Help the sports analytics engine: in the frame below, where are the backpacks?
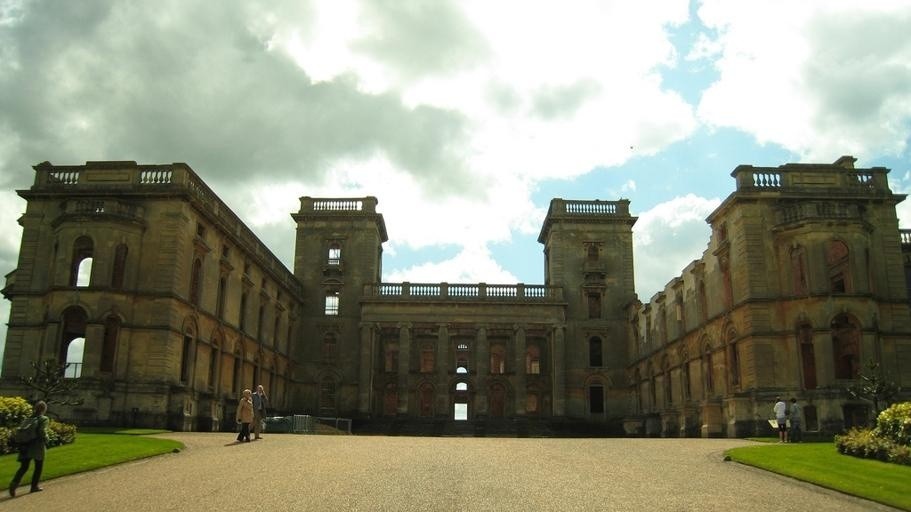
[14,415,39,443]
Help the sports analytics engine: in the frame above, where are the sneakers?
[10,482,17,497]
[31,486,45,492]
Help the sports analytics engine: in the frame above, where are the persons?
[249,384,269,439]
[236,389,254,442]
[790,396,803,442]
[8,401,52,497]
[774,396,788,443]
